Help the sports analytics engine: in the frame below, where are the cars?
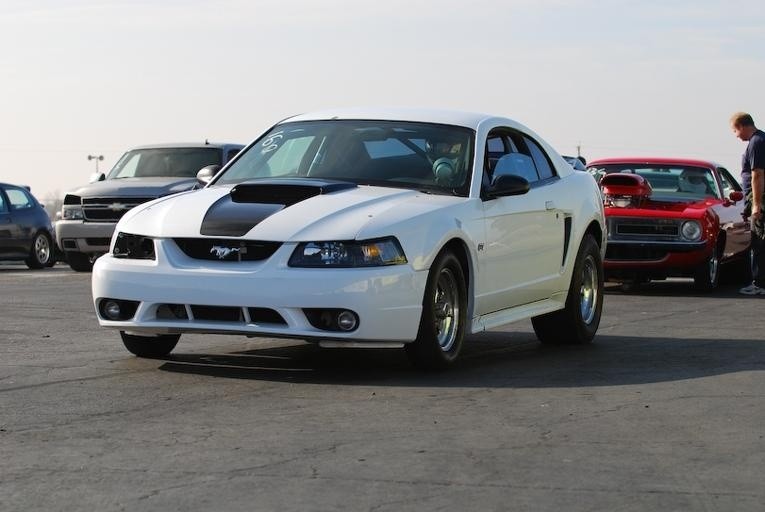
[0,182,53,269]
[55,139,251,272]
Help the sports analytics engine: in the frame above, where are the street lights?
[88,155,104,173]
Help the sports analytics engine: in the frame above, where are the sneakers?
[738,283,765,297]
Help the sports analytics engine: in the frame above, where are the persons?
[423,135,462,182]
[676,167,708,194]
[730,109,765,298]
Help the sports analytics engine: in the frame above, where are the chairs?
[676,169,717,196]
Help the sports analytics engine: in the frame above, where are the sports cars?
[91,110,610,372]
[586,156,752,289]
